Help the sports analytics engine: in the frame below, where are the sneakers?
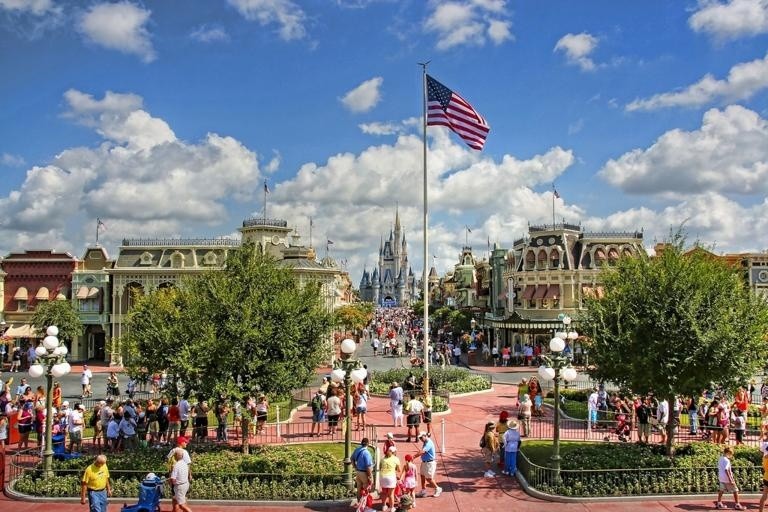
[415,492,427,497]
[485,470,515,477]
[734,503,747,510]
[361,428,366,430]
[413,502,416,507]
[382,505,388,511]
[309,431,337,437]
[355,429,359,431]
[364,507,376,512]
[427,433,431,437]
[406,438,411,442]
[416,439,419,442]
[390,507,396,512]
[434,487,442,497]
[190,437,230,445]
[634,439,667,445]
[91,443,176,448]
[716,504,727,508]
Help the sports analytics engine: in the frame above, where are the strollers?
[603,417,629,442]
[120,476,168,512]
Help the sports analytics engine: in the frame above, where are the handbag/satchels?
[479,436,486,448]
[517,412,524,420]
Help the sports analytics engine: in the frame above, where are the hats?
[498,411,508,420]
[405,454,413,462]
[79,404,85,411]
[384,432,393,438]
[389,446,396,453]
[63,401,69,406]
[417,431,426,437]
[507,420,520,429]
[259,393,266,398]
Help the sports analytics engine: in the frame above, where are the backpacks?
[89,414,98,426]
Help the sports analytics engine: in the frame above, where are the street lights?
[537,338,577,487]
[554,317,579,387]
[330,339,368,494]
[26,325,72,482]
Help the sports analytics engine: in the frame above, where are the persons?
[169,450,192,512]
[166,436,192,512]
[759,442,768,512]
[81,454,113,512]
[715,445,747,511]
[587,379,768,447]
[0,343,270,453]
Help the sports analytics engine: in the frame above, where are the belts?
[357,470,366,472]
[422,460,433,462]
[91,489,106,492]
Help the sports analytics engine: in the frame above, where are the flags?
[554,185,560,199]
[265,182,271,194]
[466,225,473,234]
[327,239,334,244]
[426,73,490,152]
[96,219,107,235]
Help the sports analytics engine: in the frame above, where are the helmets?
[400,495,413,504]
